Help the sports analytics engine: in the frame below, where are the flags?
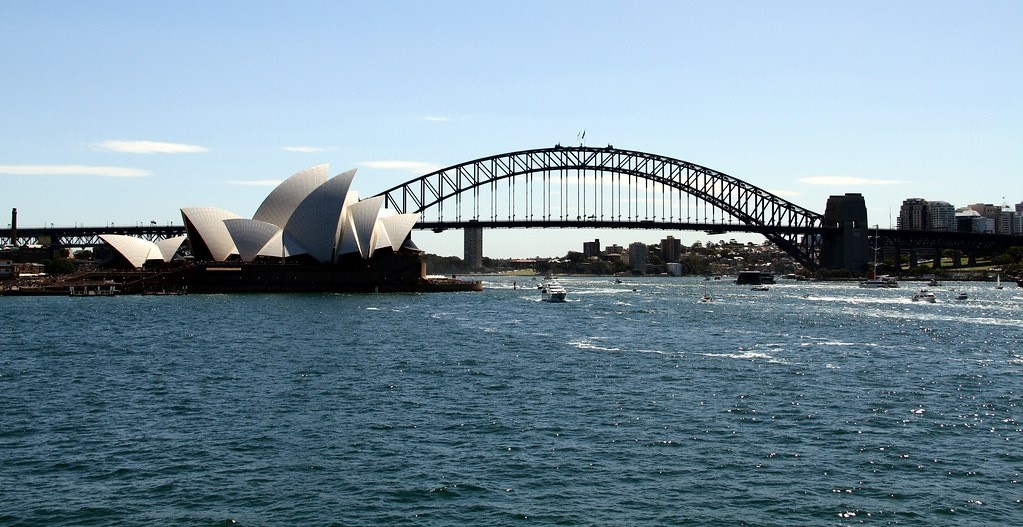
[582,130,585,139]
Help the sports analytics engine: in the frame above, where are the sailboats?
[859,224,901,288]
[995,274,1003,288]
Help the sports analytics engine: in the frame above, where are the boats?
[928,279,942,287]
[751,284,769,292]
[955,293,968,299]
[736,271,776,284]
[616,277,622,283]
[700,280,716,305]
[542,279,568,301]
[913,289,936,303]
